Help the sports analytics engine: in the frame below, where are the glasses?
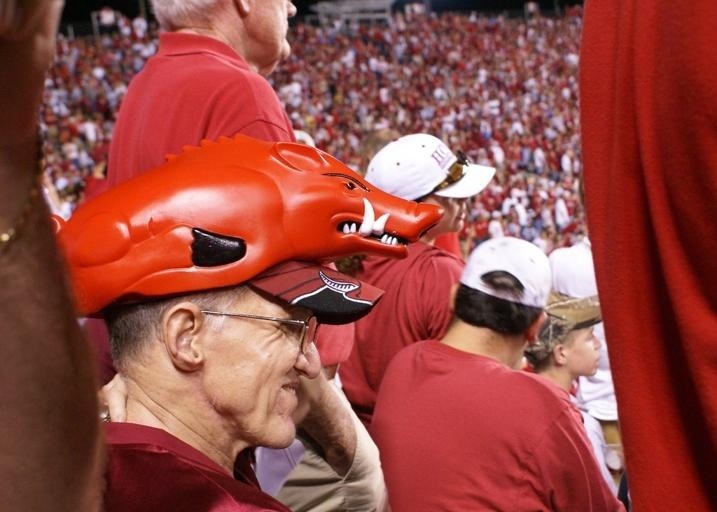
[421,149,471,201]
[202,312,321,354]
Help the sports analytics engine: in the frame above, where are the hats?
[364,132,497,201]
[249,261,385,326]
[459,236,552,308]
[524,292,602,366]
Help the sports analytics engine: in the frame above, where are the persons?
[0,0,716,512]
